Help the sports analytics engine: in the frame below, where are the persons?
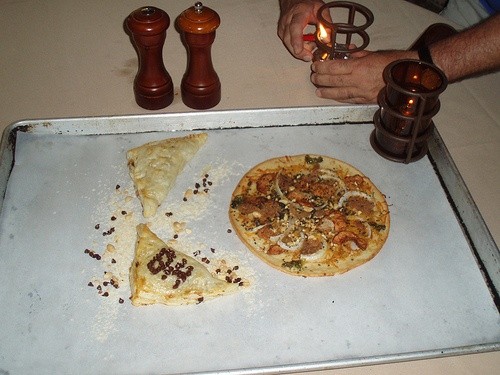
[277,0,500,104]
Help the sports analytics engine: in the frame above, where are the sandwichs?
[126,133,208,219]
[128,223,239,308]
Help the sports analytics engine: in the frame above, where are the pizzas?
[227,154,391,277]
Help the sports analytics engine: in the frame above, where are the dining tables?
[3,0,500,375]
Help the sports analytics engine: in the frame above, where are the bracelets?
[416,41,433,64]
